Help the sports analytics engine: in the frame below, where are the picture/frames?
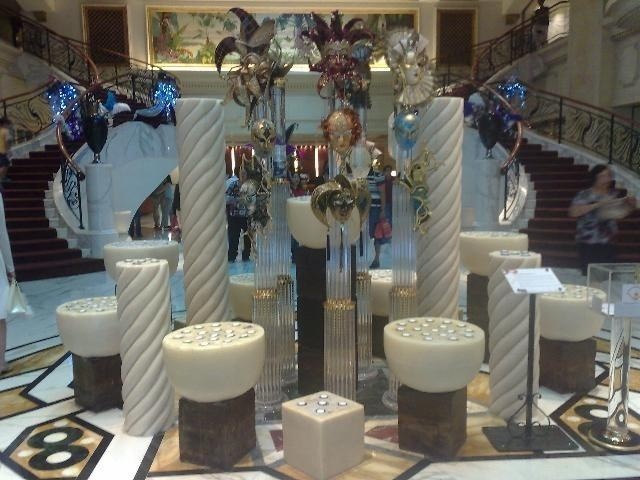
[144,5,421,68]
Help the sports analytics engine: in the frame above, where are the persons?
[0,194,15,373]
[8,0,23,49]
[130,162,394,270]
[0,155,11,181]
[567,163,634,274]
[0,118,13,154]
[530,0,549,49]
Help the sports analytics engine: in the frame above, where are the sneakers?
[369,259,382,269]
[0,365,14,375]
[153,225,179,232]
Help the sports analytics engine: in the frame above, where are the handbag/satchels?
[596,184,635,221]
[7,279,36,318]
[374,218,393,240]
